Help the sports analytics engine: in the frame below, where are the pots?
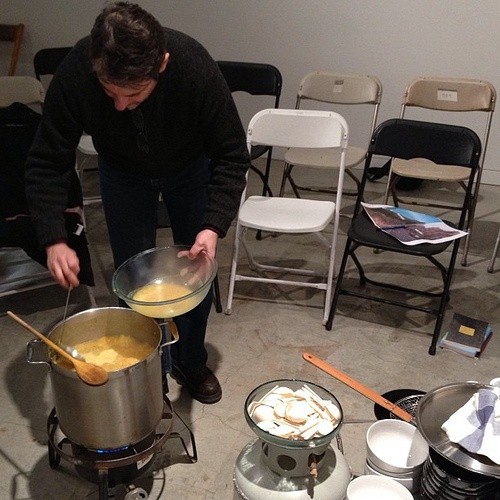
[26,308,178,451]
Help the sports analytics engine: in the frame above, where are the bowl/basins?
[244,378,345,449]
[345,419,430,500]
[113,244,218,319]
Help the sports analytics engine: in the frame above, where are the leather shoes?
[170,363,222,404]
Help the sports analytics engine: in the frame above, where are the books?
[439,313,492,360]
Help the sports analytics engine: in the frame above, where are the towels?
[441,377,500,467]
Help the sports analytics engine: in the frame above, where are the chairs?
[273,70,383,238]
[326,120,482,354]
[371,76,496,264]
[224,108,350,323]
[0,23,103,310]
[216,60,282,240]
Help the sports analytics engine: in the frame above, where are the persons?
[22,2,251,404]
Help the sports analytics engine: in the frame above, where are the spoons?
[248,382,340,442]
